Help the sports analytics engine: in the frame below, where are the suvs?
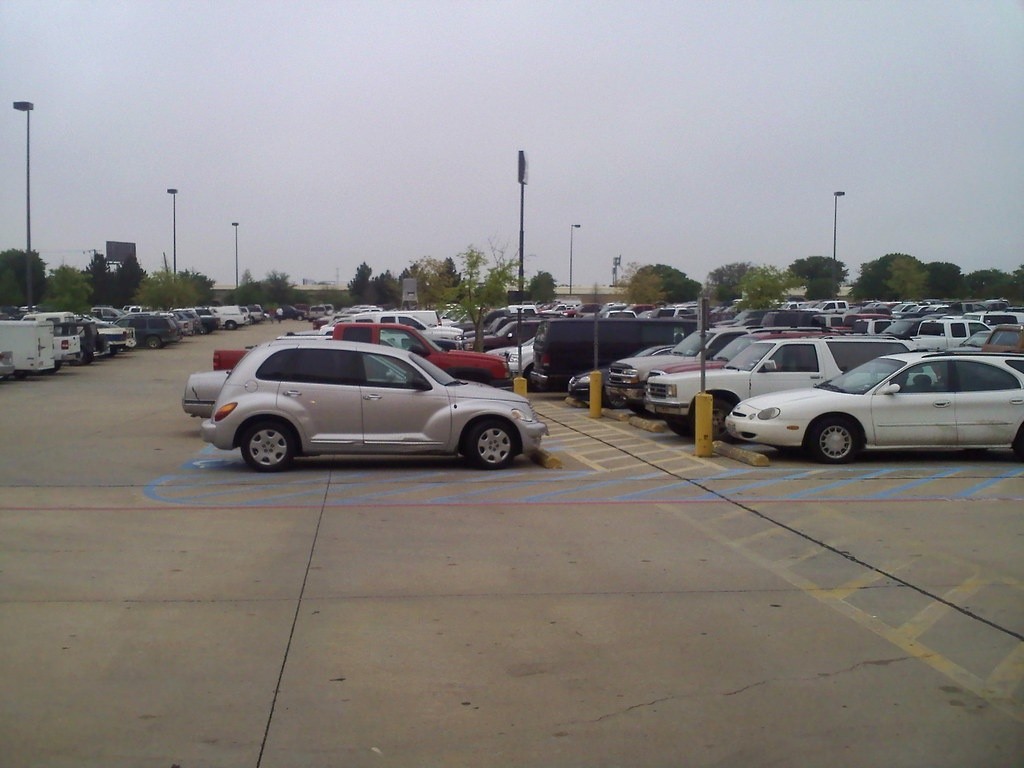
[176,297,1023,389]
[112,311,183,350]
[605,324,849,416]
[646,325,851,381]
[17,311,138,372]
[170,312,194,337]
[723,350,1024,463]
[651,328,938,443]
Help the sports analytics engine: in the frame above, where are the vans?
[91,307,126,323]
[125,305,164,314]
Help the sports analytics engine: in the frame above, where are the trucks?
[0,320,55,378]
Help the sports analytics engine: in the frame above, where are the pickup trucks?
[213,323,514,394]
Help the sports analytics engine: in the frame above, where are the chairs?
[912,374,930,386]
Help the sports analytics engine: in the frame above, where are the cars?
[182,352,418,419]
[0,350,17,376]
[567,344,677,400]
[202,338,550,473]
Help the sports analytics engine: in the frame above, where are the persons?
[269,309,275,324]
[276,306,283,324]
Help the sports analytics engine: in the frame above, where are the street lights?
[231,222,239,288]
[166,188,178,282]
[570,224,581,299]
[12,100,35,314]
[832,190,848,261]
